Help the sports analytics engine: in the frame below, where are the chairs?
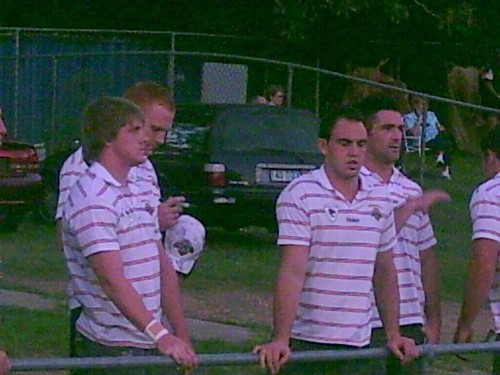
[401,129,431,158]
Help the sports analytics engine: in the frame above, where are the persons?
[364,101,452,375]
[451,124,500,375]
[55,81,200,375]
[251,106,422,375]
[402,95,455,182]
[0,108,8,150]
[254,87,286,106]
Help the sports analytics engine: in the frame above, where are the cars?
[0,139,43,234]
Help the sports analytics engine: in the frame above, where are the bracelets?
[144,317,170,344]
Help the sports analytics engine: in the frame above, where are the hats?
[164,215,205,274]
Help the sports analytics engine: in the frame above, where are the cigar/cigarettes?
[168,196,190,208]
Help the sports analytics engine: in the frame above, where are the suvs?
[37,104,326,238]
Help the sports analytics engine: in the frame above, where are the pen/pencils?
[161,198,197,208]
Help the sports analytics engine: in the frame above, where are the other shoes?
[436,160,444,168]
[443,176,452,180]
